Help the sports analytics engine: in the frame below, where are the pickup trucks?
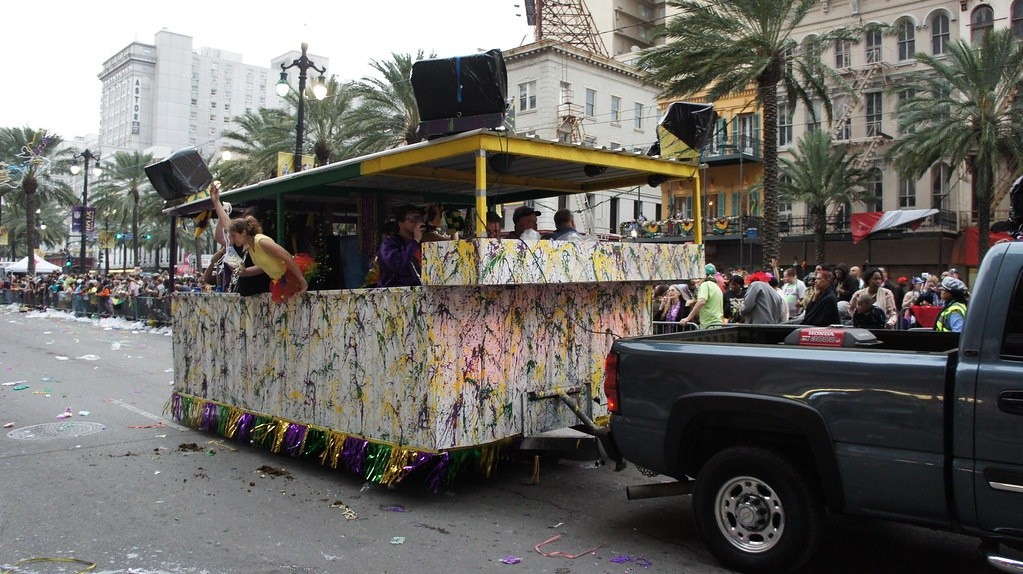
[602,236,1022,574]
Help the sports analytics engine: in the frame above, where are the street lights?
[275,40,327,173]
[70,148,102,274]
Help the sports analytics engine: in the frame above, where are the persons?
[653,259,969,333]
[204,183,582,300]
[676,208,683,219]
[0,270,204,327]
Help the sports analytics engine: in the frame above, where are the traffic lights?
[140,234,151,239]
[98,250,103,263]
[114,234,125,238]
[67,255,71,266]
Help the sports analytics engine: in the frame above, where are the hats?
[911,277,924,284]
[898,276,908,284]
[938,276,969,291]
[486,211,503,223]
[513,206,541,224]
[705,263,716,274]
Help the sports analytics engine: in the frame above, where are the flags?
[194,210,210,239]
[851,209,940,245]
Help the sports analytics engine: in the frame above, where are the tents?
[5,253,62,274]
